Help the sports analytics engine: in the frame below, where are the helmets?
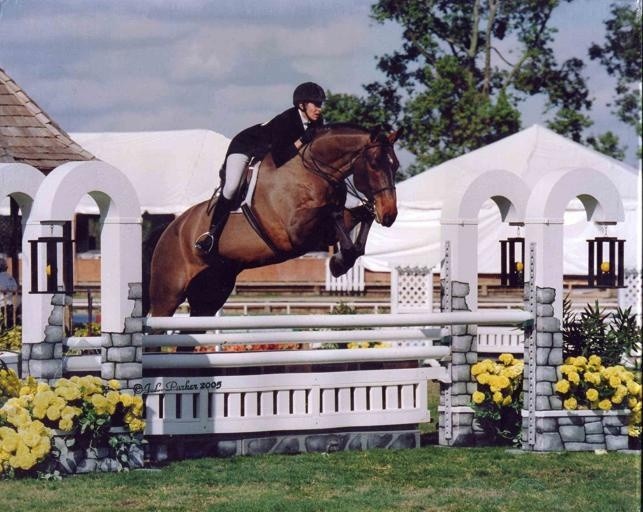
[293,81,327,105]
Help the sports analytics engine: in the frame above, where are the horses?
[143,122,407,355]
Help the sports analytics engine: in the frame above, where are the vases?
[9,449,39,481]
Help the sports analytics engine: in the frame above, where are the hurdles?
[0,310,535,474]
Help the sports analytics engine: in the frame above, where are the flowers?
[554,353,643,442]
[471,352,525,429]
[0,374,145,471]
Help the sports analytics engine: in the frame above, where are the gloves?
[301,126,315,143]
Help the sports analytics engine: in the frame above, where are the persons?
[0,258,18,292]
[192,83,328,260]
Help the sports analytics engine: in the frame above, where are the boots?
[195,190,233,256]
